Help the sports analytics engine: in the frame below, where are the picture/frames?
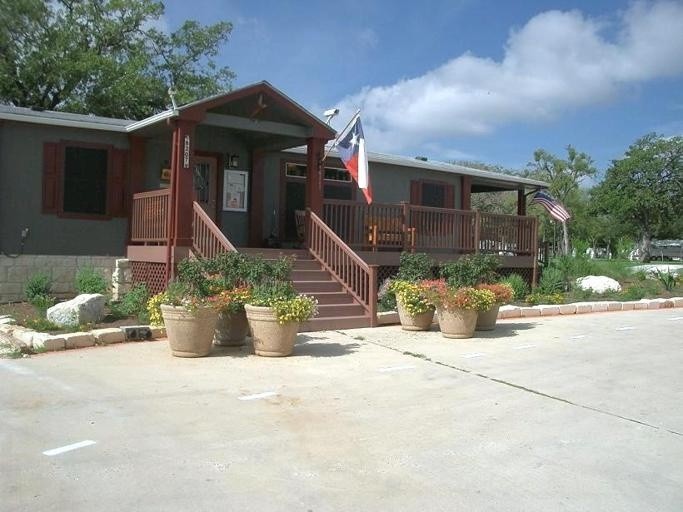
[222,170,247,213]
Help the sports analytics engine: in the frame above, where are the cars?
[479,240,513,256]
[586,243,611,260]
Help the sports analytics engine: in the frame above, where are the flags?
[333,115,372,207]
[528,191,571,223]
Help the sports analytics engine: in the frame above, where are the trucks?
[628,240,683,262]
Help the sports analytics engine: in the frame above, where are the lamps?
[224,152,239,169]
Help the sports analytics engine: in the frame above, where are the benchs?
[363,213,416,251]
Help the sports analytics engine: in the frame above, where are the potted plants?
[378,249,515,337]
[146,250,319,359]
